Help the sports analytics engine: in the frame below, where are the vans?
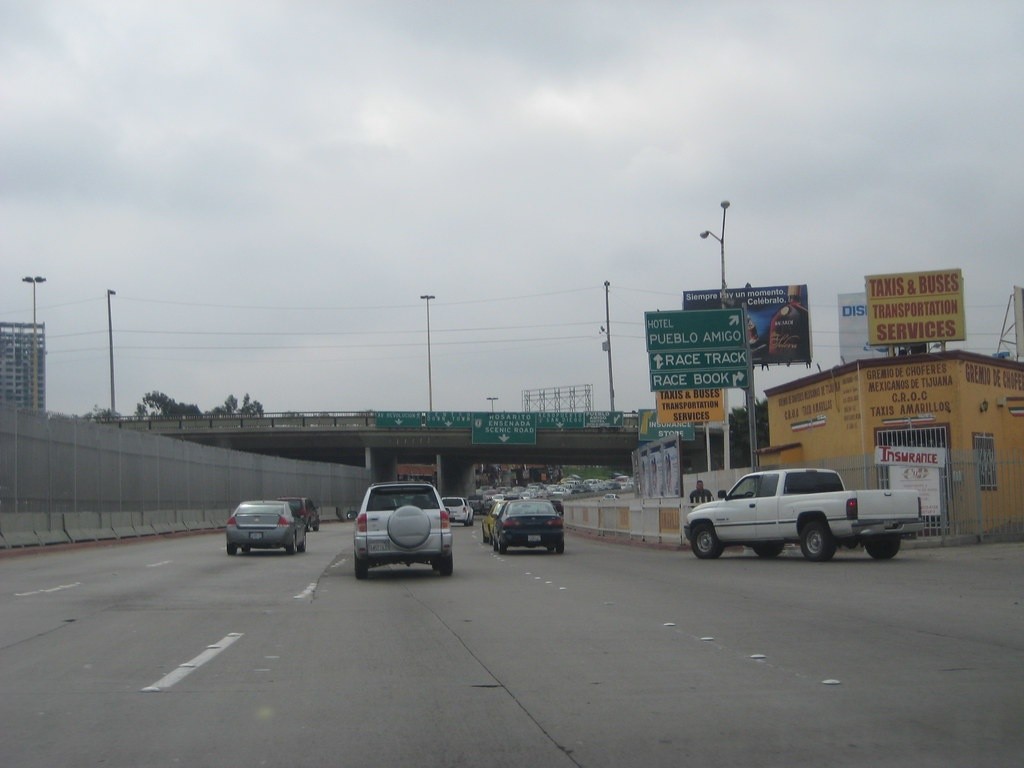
[275,497,320,532]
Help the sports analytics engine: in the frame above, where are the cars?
[224,501,309,555]
[481,501,513,545]
[467,472,636,515]
[489,499,566,554]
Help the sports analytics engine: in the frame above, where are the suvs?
[352,481,453,580]
[441,496,475,526]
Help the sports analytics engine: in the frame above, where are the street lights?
[23,275,47,411]
[420,294,435,411]
[600,280,616,411]
[699,199,730,308]
[107,289,117,415]
[487,397,498,412]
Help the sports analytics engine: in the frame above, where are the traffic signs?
[537,412,584,429]
[585,411,623,428]
[650,371,749,392]
[648,348,751,372]
[472,411,537,445]
[376,411,422,428]
[645,308,746,351]
[426,410,472,428]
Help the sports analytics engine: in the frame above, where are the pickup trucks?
[684,467,925,561]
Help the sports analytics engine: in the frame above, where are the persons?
[689,481,717,508]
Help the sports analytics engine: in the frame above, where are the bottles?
[741,303,759,344]
[768,285,809,362]
